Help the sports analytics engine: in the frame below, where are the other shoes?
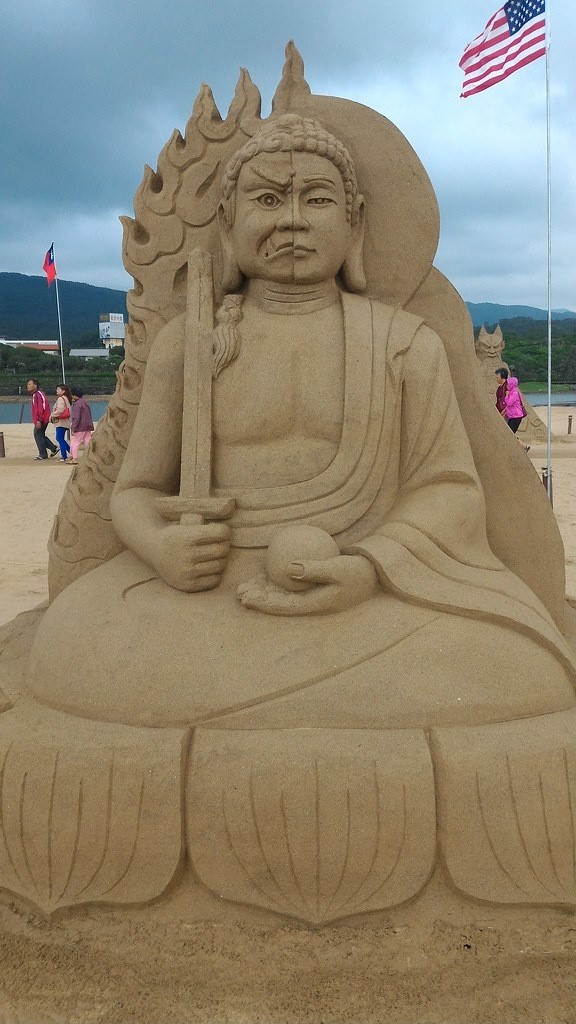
[50,446,60,457]
[57,458,68,461]
[66,459,78,464]
[67,452,72,457]
[34,455,47,460]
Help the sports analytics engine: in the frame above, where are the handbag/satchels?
[54,396,70,419]
[516,389,527,418]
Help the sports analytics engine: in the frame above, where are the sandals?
[524,445,530,453]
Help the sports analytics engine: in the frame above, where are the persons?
[26,379,59,460]
[477,324,511,393]
[66,386,95,464]
[48,383,72,462]
[493,367,531,453]
[36,114,576,727]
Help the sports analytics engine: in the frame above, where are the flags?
[458,0,547,98]
[42,243,56,287]
